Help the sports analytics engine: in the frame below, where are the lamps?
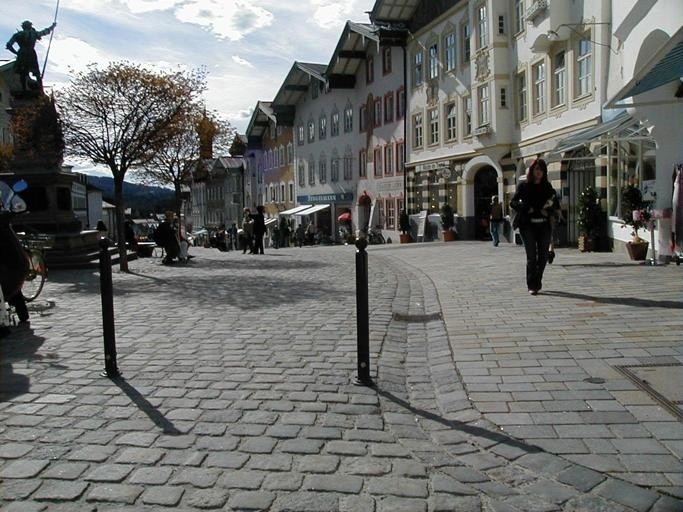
[546,21,612,49]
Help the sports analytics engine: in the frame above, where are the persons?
[546,181,563,265]
[123,220,136,252]
[3,17,58,95]
[160,210,187,265]
[486,195,503,248]
[399,207,411,235]
[196,203,351,254]
[510,158,560,297]
[172,216,196,261]
[95,220,107,231]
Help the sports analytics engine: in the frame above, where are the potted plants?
[397,211,412,243]
[626,205,654,240]
[577,183,601,252]
[441,203,456,242]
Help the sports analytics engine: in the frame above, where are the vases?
[627,236,649,261]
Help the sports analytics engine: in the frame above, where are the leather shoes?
[528,289,538,296]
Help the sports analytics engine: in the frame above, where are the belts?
[531,218,547,223]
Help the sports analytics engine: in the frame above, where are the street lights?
[239,162,244,220]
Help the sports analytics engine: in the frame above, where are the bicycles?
[16,232,55,301]
[363,227,386,244]
